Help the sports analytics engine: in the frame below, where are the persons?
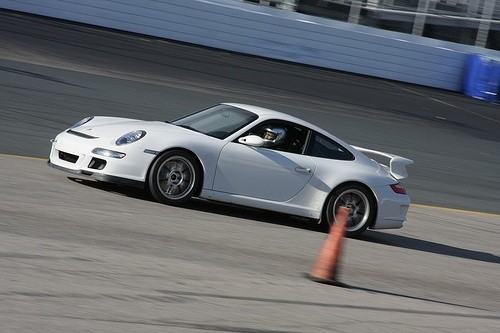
[261,126,286,151]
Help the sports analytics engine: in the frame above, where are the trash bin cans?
[460,52,500,103]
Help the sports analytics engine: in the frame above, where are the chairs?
[277,130,308,153]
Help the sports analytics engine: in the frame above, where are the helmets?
[263,124,286,143]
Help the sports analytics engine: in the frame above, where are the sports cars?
[46,100,414,238]
